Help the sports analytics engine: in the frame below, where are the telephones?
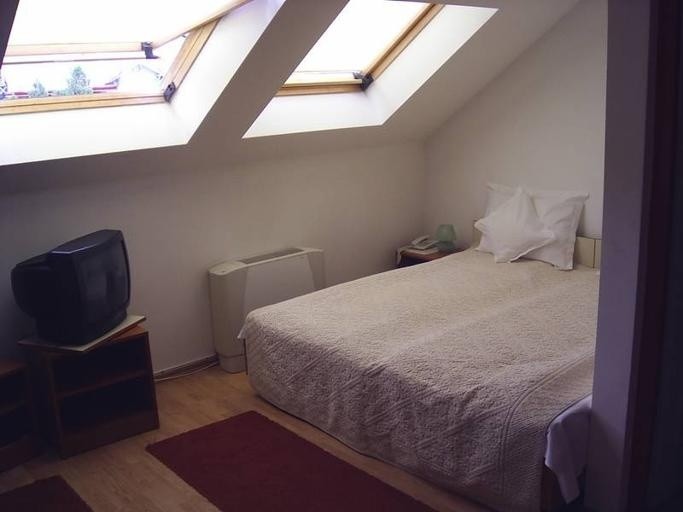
[411,235,440,250]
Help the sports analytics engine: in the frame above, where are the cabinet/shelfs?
[0,329,161,478]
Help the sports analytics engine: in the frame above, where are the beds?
[242,234,603,512]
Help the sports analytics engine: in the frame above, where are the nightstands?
[395,247,464,267]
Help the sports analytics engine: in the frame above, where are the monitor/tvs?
[10,229,131,347]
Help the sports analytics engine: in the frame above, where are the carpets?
[0,472,95,512]
[146,408,435,512]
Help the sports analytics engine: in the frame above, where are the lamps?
[436,224,458,252]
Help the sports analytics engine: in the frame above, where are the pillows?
[473,176,593,273]
